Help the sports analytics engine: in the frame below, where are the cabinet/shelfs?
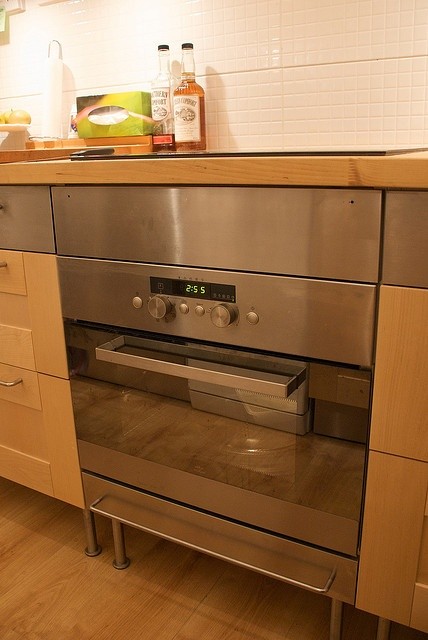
[353,281,428,632]
[0,248,86,511]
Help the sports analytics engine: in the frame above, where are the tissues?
[75,91,150,139]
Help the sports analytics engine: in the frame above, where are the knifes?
[28,148,114,162]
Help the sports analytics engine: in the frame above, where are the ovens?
[52,256,381,608]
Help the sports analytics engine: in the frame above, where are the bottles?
[151,45,178,154]
[174,43,207,154]
